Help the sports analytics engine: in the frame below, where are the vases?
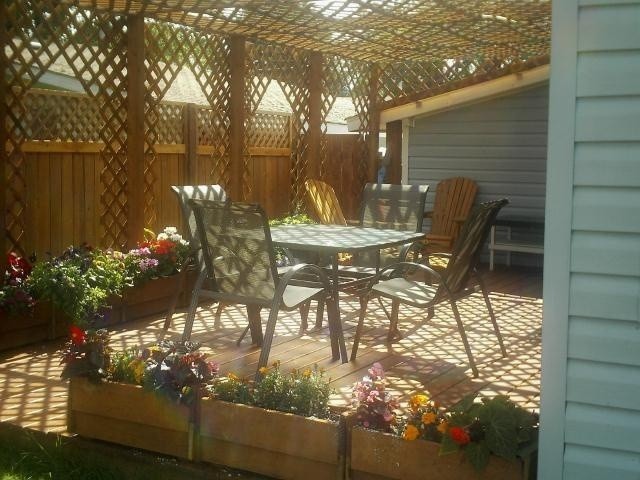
[0,273,181,351]
[199,397,345,480]
[350,426,522,480]
[65,376,195,462]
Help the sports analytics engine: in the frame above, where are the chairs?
[155,184,310,347]
[349,199,511,381]
[305,179,360,267]
[180,198,341,385]
[316,182,430,328]
[403,176,479,293]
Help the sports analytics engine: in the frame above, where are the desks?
[220,223,425,334]
[489,214,545,270]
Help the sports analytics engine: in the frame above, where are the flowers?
[344,362,532,477]
[203,359,339,422]
[58,328,218,408]
[0,226,188,327]
